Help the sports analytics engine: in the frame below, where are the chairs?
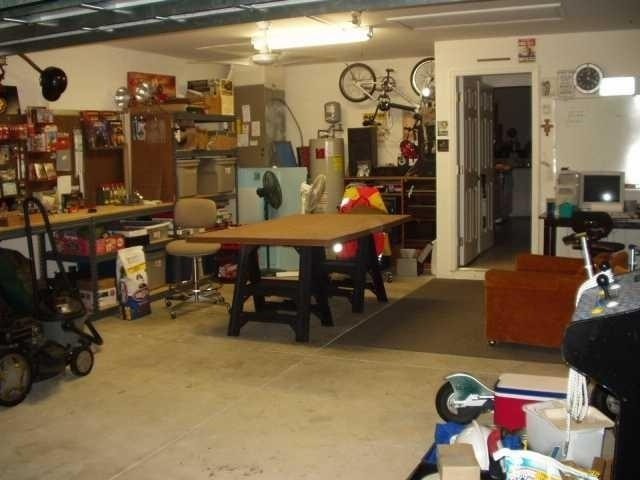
[166,197,231,319]
[484,252,629,350]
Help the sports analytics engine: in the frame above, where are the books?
[116,256,143,296]
[118,244,147,292]
[80,110,125,149]
[493,373,566,426]
[24,105,58,183]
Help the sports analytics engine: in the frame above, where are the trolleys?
[0,193,102,408]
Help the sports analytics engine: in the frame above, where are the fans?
[257,170,282,277]
[184,21,337,66]
[276,174,327,277]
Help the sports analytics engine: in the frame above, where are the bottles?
[98,182,127,204]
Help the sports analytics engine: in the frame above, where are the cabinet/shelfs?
[130,111,241,225]
[501,167,530,217]
[0,198,174,321]
[0,112,87,214]
[345,175,436,270]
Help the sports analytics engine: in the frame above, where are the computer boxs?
[559,173,581,208]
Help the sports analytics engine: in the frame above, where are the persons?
[119,282,127,304]
[136,274,147,292]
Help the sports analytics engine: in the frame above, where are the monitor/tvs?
[578,170,625,213]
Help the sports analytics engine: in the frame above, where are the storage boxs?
[54,218,171,311]
[185,77,235,115]
[193,156,237,195]
[176,158,200,197]
[493,372,615,469]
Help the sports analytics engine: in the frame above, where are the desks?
[538,209,640,256]
[185,212,412,343]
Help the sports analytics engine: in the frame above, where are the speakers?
[347,127,378,176]
[397,156,409,176]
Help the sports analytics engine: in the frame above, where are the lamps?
[114,81,165,112]
[249,22,373,51]
[16,53,68,101]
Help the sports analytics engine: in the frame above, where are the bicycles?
[338,56,435,127]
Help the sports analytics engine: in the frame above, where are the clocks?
[572,62,603,94]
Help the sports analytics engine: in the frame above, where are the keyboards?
[608,213,633,219]
